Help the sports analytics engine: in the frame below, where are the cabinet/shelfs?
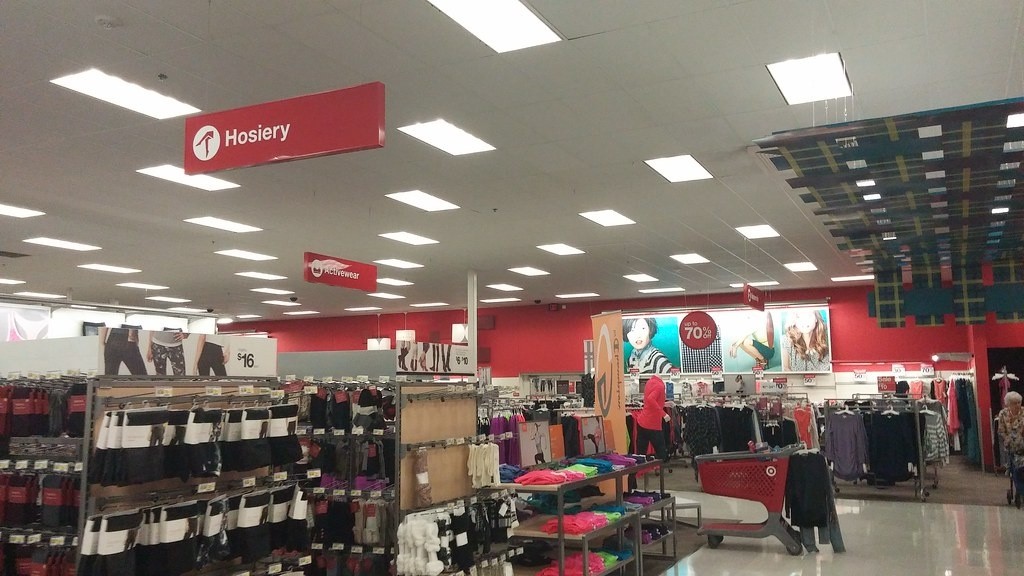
[500,451,677,576]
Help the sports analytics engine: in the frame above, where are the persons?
[103,328,147,375]
[997,391,1024,509]
[730,311,775,370]
[735,375,744,397]
[622,318,675,373]
[193,334,230,377]
[397,341,452,372]
[529,423,547,464]
[783,309,830,372]
[147,330,191,376]
[635,374,671,462]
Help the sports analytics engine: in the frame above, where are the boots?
[436,346,439,371]
[430,345,435,371]
[401,348,408,371]
[398,347,408,367]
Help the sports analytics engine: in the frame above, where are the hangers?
[624,360,1020,418]
[792,440,821,460]
[478,394,584,432]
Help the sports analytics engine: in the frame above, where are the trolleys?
[694,444,807,558]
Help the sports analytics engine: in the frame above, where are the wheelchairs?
[993,419,1024,507]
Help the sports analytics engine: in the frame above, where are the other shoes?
[410,359,415,370]
[446,357,451,371]
[415,360,417,370]
[443,357,447,372]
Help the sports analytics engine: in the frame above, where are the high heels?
[419,358,428,371]
[752,357,769,370]
